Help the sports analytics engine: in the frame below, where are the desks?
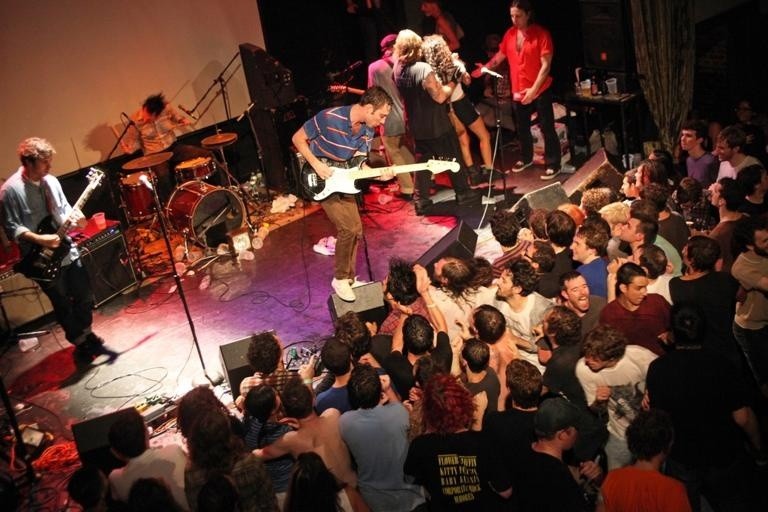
[564,90,643,170]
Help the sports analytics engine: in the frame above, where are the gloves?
[450,66,463,85]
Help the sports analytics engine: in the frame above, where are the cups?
[581,81,591,97]
[93,213,106,230]
[186,252,200,263]
[606,80,618,94]
[379,194,392,204]
[239,248,255,262]
[258,227,269,240]
[561,163,575,174]
[20,337,39,352]
[175,262,184,277]
[173,245,188,260]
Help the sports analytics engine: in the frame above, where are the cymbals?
[121,152,172,169]
[201,133,238,150]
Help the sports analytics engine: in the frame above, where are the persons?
[420,1,465,54]
[390,28,485,217]
[422,34,506,185]
[1,104,768,512]
[475,33,516,134]
[291,82,396,304]
[367,32,417,201]
[122,89,193,201]
[471,2,562,182]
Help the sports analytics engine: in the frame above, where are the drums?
[164,179,247,251]
[175,158,216,181]
[118,171,158,220]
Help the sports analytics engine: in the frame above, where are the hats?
[534,397,594,432]
[380,34,397,52]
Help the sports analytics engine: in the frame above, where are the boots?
[486,169,508,182]
[467,164,489,186]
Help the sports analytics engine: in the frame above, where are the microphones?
[236,98,258,122]
[123,112,142,135]
[481,66,503,79]
[139,175,166,207]
[178,103,197,120]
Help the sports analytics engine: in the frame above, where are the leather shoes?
[456,188,481,205]
[414,197,434,211]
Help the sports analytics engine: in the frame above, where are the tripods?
[238,186,262,232]
[258,152,282,203]
[0,294,50,359]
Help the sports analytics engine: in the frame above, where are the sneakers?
[512,160,533,173]
[539,168,560,180]
[350,276,375,289]
[331,277,357,302]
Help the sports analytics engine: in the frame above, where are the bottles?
[591,76,598,96]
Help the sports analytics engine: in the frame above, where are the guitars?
[301,154,460,203]
[21,167,105,284]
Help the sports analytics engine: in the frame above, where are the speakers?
[412,220,477,273]
[71,407,150,468]
[327,281,388,330]
[509,181,572,230]
[217,329,275,403]
